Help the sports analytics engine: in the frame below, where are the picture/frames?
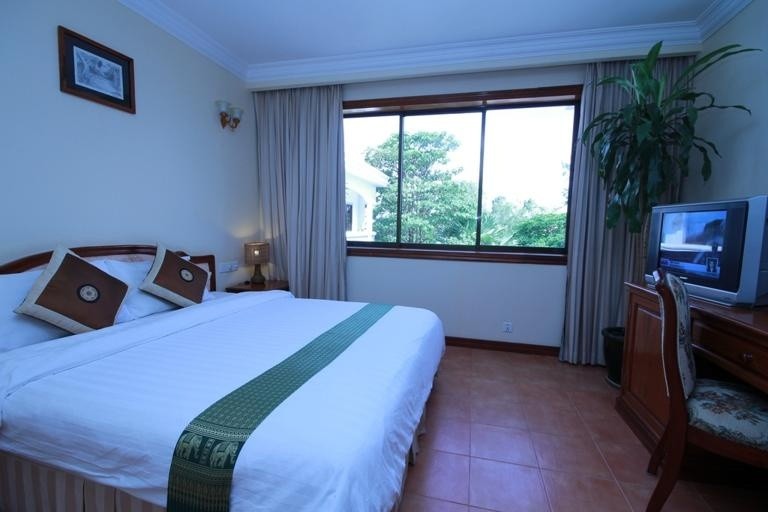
[56,23,136,116]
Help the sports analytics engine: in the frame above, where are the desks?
[613,278,766,512]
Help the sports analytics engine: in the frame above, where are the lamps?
[245,242,270,284]
[216,98,245,129]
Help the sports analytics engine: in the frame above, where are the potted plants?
[579,38,767,389]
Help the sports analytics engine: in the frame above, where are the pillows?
[0,239,261,352]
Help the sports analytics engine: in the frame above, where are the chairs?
[647,268,767,512]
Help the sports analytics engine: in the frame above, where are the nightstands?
[225,279,289,293]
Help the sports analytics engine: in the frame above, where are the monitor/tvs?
[642,195,768,309]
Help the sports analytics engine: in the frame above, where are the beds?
[0,247,448,509]
